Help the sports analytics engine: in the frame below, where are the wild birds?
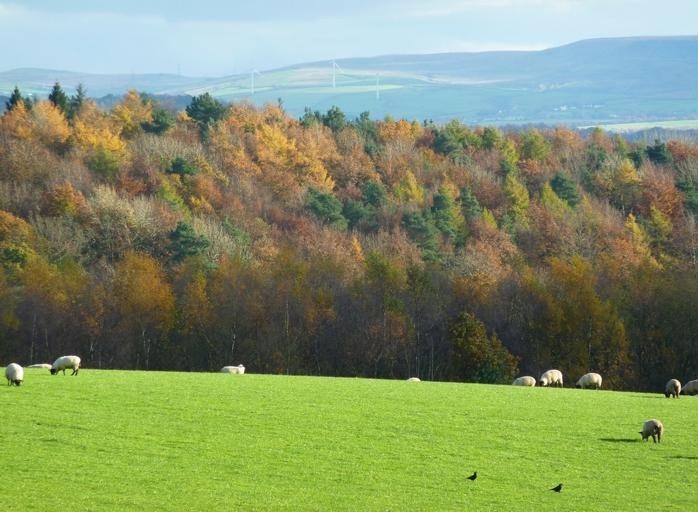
[550,483,563,493]
[466,472,477,481]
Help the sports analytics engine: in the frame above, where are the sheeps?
[539,369,564,388]
[48,355,81,376]
[575,372,603,389]
[5,362,23,386]
[220,364,246,374]
[680,379,698,395]
[27,363,52,370]
[512,376,536,387]
[662,378,682,398]
[638,419,663,443]
